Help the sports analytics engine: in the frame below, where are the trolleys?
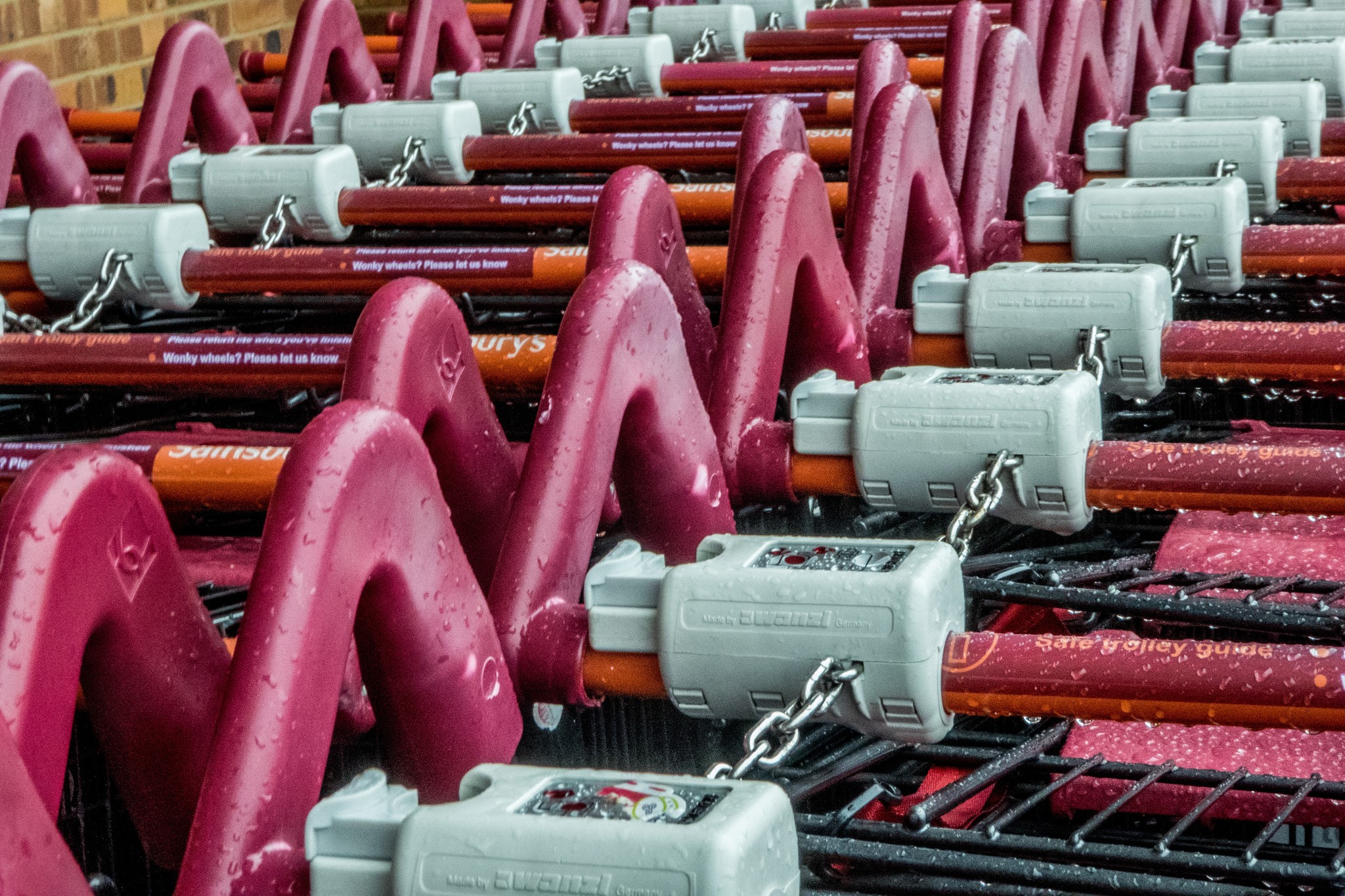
[0,0,1345,896]
